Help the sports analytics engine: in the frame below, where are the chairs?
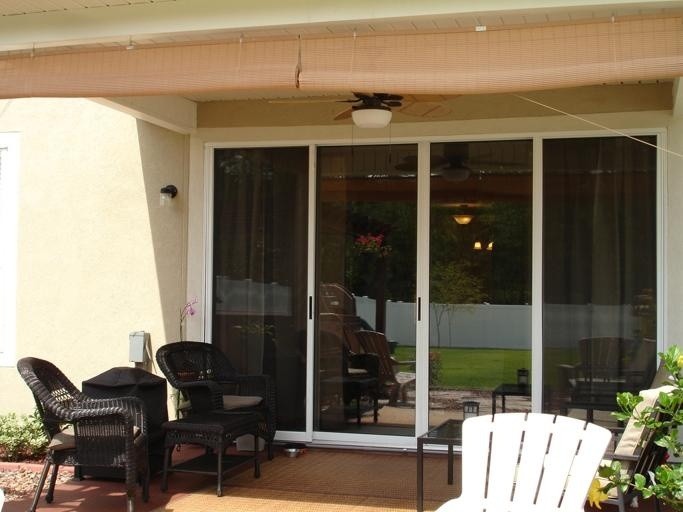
[555,337,642,428]
[16,356,151,512]
[354,330,416,403]
[156,341,277,459]
[587,385,682,512]
[275,328,380,429]
[436,412,613,512]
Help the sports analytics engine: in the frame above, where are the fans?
[266,91,463,120]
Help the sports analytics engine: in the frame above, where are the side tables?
[161,411,260,497]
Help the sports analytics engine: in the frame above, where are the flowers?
[349,230,393,262]
[587,342,682,512]
[177,296,200,341]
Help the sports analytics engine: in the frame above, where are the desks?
[491,383,550,415]
[416,418,466,512]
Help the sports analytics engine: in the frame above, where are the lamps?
[350,99,392,128]
[451,204,475,224]
